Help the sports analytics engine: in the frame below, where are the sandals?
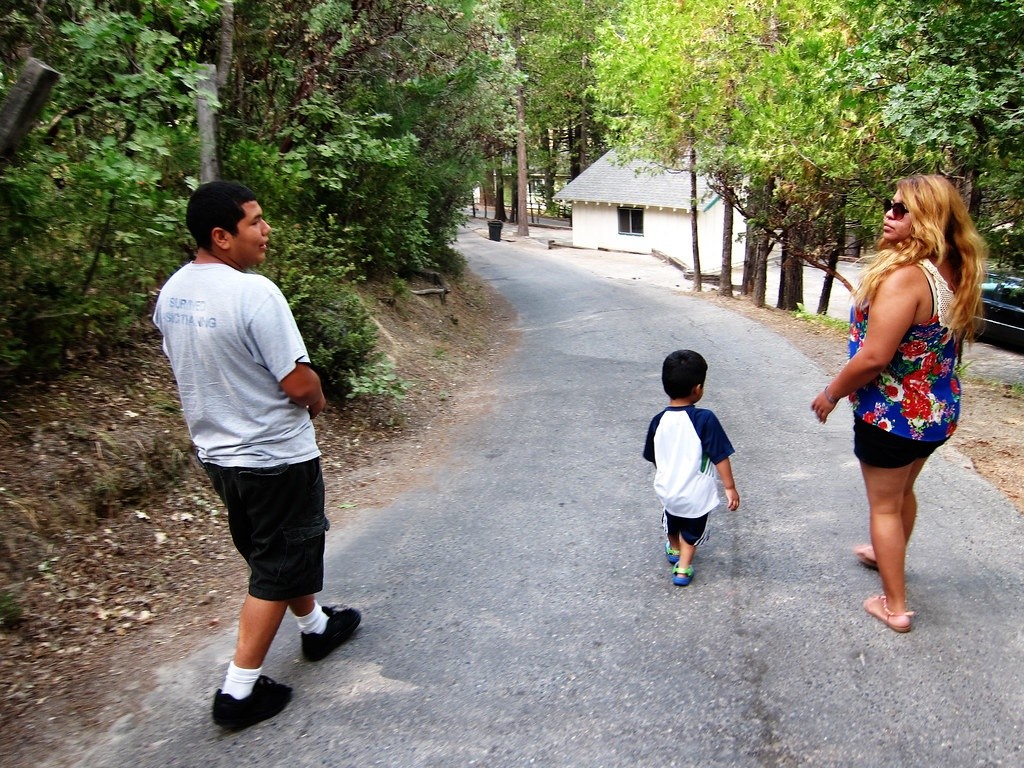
[855,545,879,569]
[865,596,913,633]
[665,540,680,562]
[673,561,694,586]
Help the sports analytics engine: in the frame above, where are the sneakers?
[212,675,292,730]
[301,606,361,662]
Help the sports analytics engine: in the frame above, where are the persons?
[809,174,987,632]
[642,349,739,586]
[151,181,361,732]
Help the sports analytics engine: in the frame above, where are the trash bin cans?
[487,220,504,241]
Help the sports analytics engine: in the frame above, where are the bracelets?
[824,385,840,404]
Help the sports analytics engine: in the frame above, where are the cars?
[975,271,1024,347]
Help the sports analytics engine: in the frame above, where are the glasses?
[882,198,909,220]
